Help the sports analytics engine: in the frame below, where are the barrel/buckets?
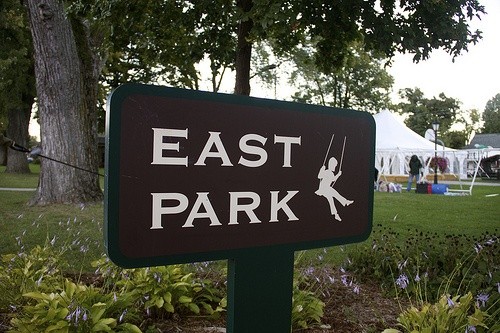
[427,184,447,194]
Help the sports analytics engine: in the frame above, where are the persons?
[406,154,422,192]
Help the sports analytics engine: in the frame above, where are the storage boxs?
[415,182,432,194]
[431,183,449,194]
